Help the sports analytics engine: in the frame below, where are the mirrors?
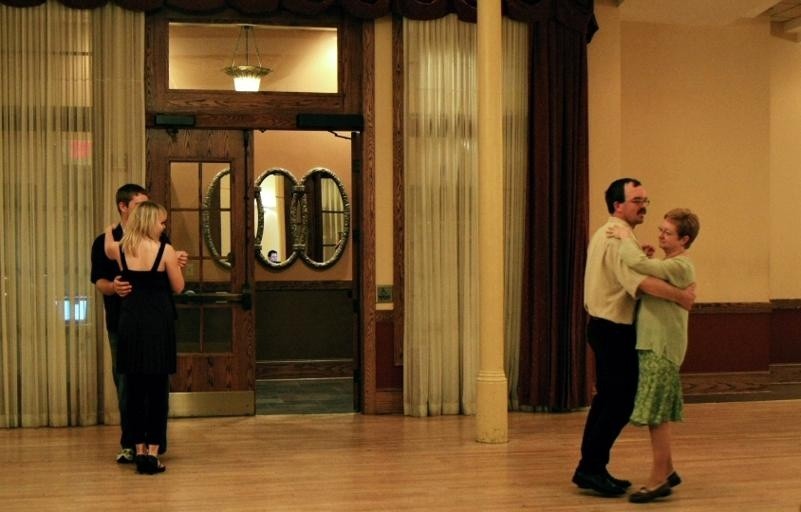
[200,167,351,273]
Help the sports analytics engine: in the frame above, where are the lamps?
[218,24,273,92]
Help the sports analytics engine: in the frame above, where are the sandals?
[136,454,166,475]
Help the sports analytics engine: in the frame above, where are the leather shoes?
[630,470,682,503]
[571,468,632,497]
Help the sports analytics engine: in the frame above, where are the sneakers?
[116,448,134,462]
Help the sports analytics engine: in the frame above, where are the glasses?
[624,199,650,207]
[658,227,678,236]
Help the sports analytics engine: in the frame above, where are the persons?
[90,182,189,464]
[605,207,701,503]
[570,177,697,498]
[268,250,278,262]
[103,201,189,475]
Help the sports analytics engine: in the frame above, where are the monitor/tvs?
[54,297,91,324]
